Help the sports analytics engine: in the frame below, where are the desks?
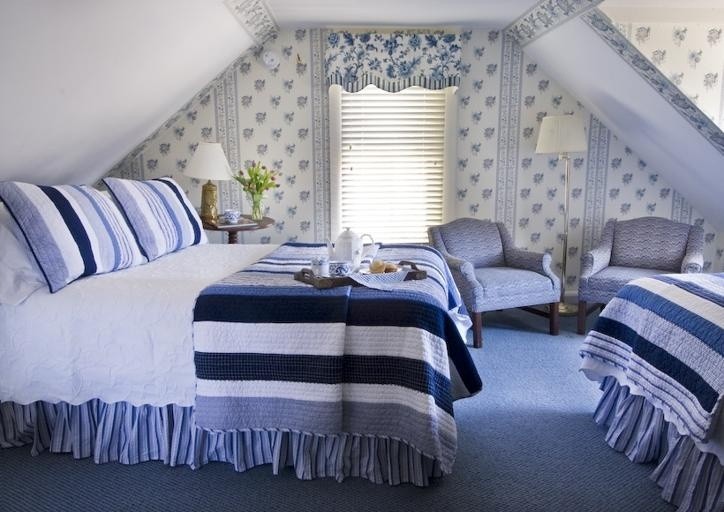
[191,212,275,243]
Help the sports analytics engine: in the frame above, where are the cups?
[309,256,328,279]
[224,209,242,225]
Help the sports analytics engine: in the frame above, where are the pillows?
[0,175,207,308]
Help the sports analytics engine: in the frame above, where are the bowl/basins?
[328,263,352,278]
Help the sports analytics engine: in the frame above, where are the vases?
[251,192,261,221]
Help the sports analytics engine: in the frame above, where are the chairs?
[574,216,705,336]
[423,216,563,348]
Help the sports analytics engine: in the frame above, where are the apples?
[369,260,385,273]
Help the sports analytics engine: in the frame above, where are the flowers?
[230,160,282,219]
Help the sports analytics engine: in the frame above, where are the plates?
[212,217,260,229]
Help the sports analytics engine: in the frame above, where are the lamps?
[184,142,236,228]
[534,115,592,317]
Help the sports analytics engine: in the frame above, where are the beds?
[0,180,484,491]
[578,272,724,510]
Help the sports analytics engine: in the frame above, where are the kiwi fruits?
[385,263,398,272]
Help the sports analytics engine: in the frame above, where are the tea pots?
[324,225,375,272]
